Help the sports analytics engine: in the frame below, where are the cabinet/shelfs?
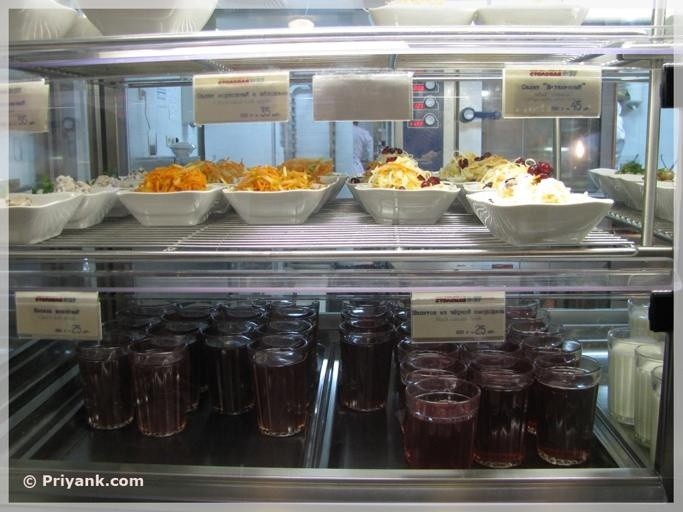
[9,9,673,502]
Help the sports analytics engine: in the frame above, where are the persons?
[353,121,374,177]
[615,99,625,170]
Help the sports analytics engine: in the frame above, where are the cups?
[73,296,665,469]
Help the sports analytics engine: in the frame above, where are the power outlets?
[137,86,146,101]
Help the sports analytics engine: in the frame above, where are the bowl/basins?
[8,6,214,43]
[168,146,196,157]
[8,168,674,247]
[361,1,585,28]
